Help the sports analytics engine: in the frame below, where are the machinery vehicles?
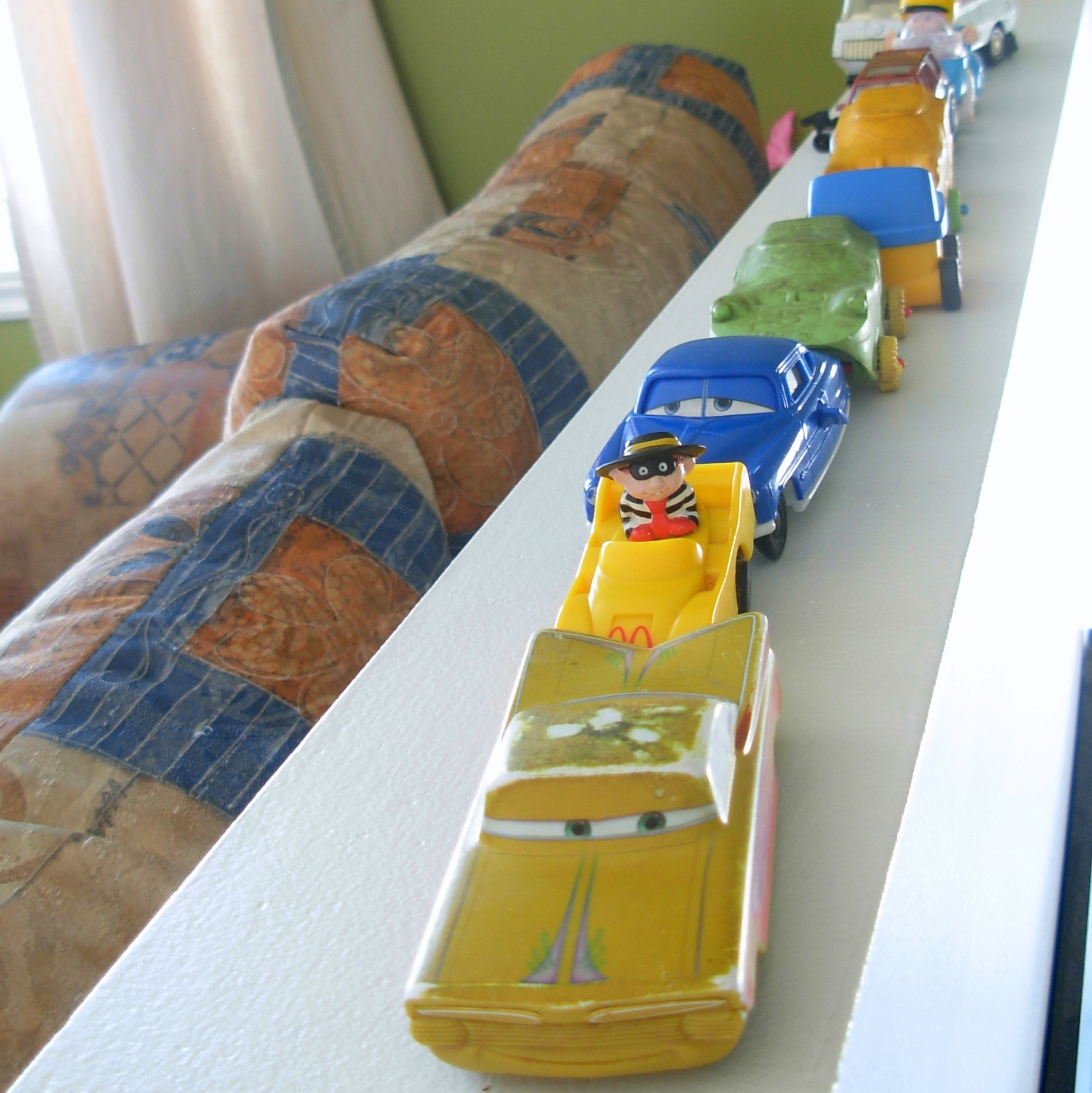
[823,86,956,193]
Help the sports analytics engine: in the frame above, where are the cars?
[831,0,1017,84]
[704,214,906,397]
[583,333,855,562]
[836,49,960,141]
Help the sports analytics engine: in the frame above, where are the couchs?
[1,42,764,1092]
